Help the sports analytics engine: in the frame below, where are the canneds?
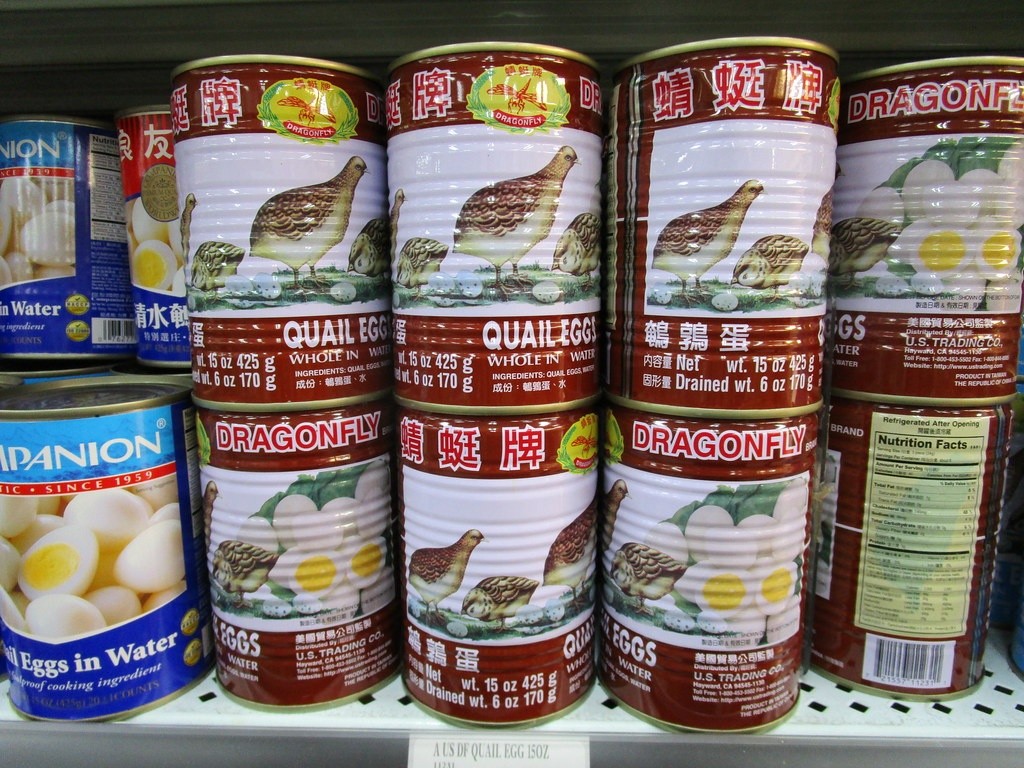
[114,103,190,367]
[2,363,110,383]
[990,377,1024,630]
[169,53,390,413]
[599,405,827,736]
[0,374,216,726]
[0,116,136,359]
[808,396,1012,703]
[383,41,604,414]
[600,37,839,420]
[394,402,599,732]
[1009,578,1023,680]
[826,55,1024,406]
[194,400,403,712]
